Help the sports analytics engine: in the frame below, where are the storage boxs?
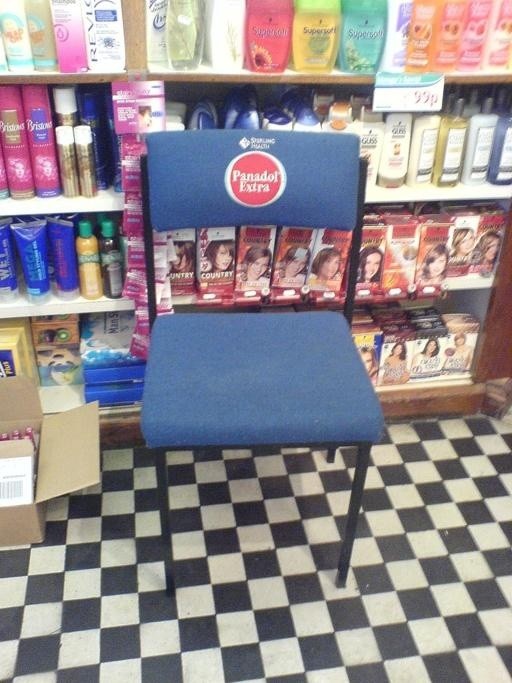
[0,375,101,547]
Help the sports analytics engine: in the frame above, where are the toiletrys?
[145,1,512,193]
[0,1,126,200]
[1,214,81,300]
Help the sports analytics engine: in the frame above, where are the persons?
[170,240,341,290]
[358,333,473,382]
[356,247,385,283]
[415,229,502,287]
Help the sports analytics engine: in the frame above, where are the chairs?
[139,129,385,596]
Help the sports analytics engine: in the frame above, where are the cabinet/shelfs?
[0,74,512,444]
